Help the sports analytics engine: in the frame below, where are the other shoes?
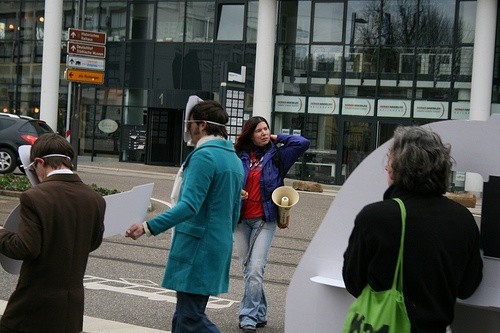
[238,323,256,333]
[256,321,267,328]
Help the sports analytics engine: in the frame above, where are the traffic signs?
[67,28,107,45]
[66,54,105,71]
[64,68,105,85]
[66,41,106,59]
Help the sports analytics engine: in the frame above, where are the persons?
[125,101,243,333]
[233,117,310,333]
[0,132,106,333]
[342,126,483,333]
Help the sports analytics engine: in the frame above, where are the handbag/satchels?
[342,283,411,333]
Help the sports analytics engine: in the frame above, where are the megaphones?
[272,186,299,229]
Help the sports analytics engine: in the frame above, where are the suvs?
[0,112,55,174]
